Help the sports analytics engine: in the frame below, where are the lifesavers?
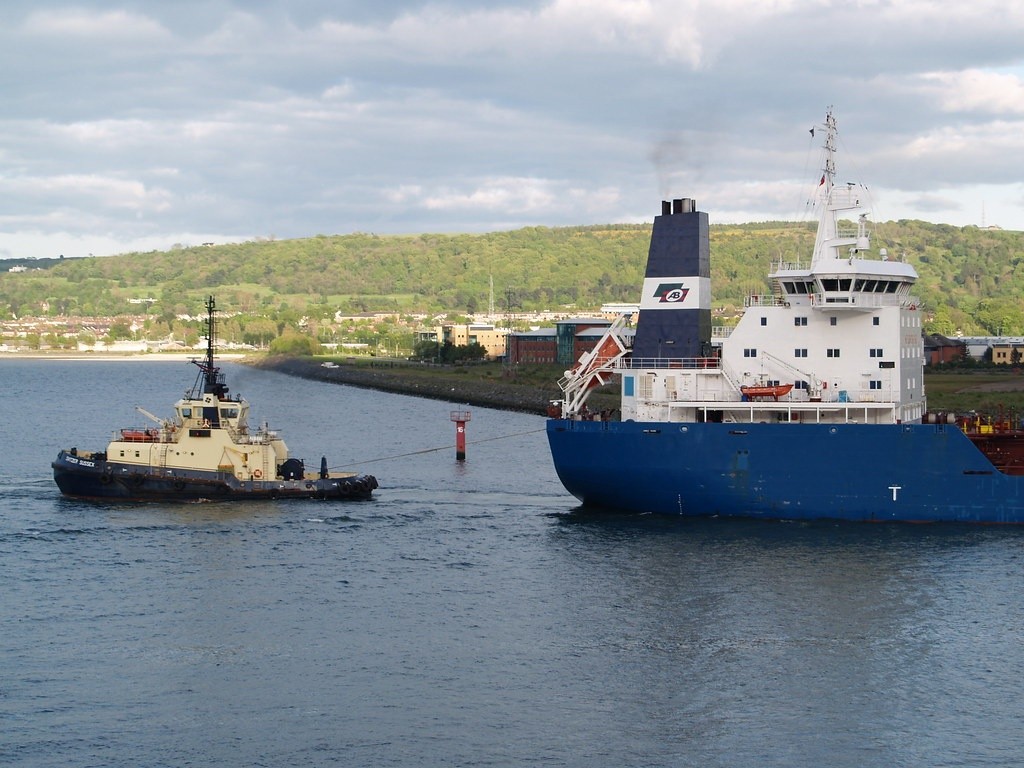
[254,469,262,477]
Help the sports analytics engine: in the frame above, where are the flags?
[809,128,814,137]
[819,174,825,186]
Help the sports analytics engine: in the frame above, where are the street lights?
[342,337,390,357]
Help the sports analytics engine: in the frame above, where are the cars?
[321,362,339,368]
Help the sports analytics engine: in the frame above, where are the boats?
[51,294,378,503]
[544,108,1024,525]
